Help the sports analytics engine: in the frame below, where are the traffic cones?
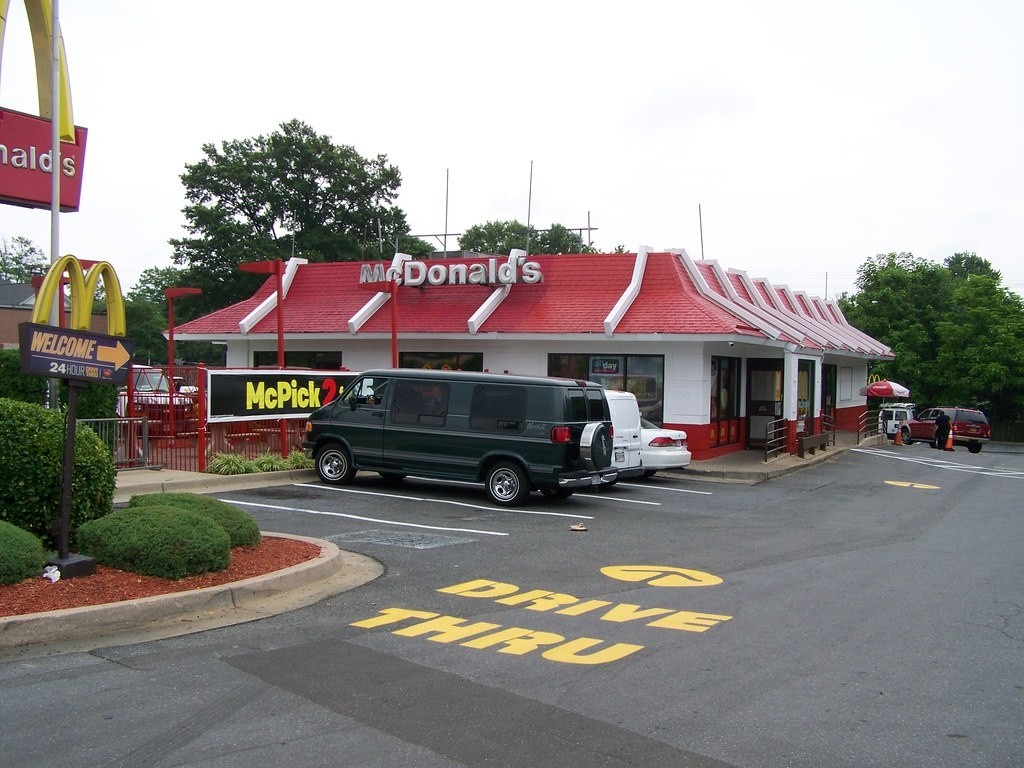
[944,429,956,451]
[892,427,904,446]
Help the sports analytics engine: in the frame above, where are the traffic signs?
[20,320,136,387]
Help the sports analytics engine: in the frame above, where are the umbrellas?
[859,378,910,409]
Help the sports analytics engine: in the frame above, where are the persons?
[935,411,952,450]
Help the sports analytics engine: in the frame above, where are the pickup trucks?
[116,365,199,419]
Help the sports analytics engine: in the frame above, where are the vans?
[900,406,990,454]
[298,371,640,508]
[877,403,916,439]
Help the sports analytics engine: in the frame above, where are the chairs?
[396,391,426,415]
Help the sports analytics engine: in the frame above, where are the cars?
[639,417,692,477]
